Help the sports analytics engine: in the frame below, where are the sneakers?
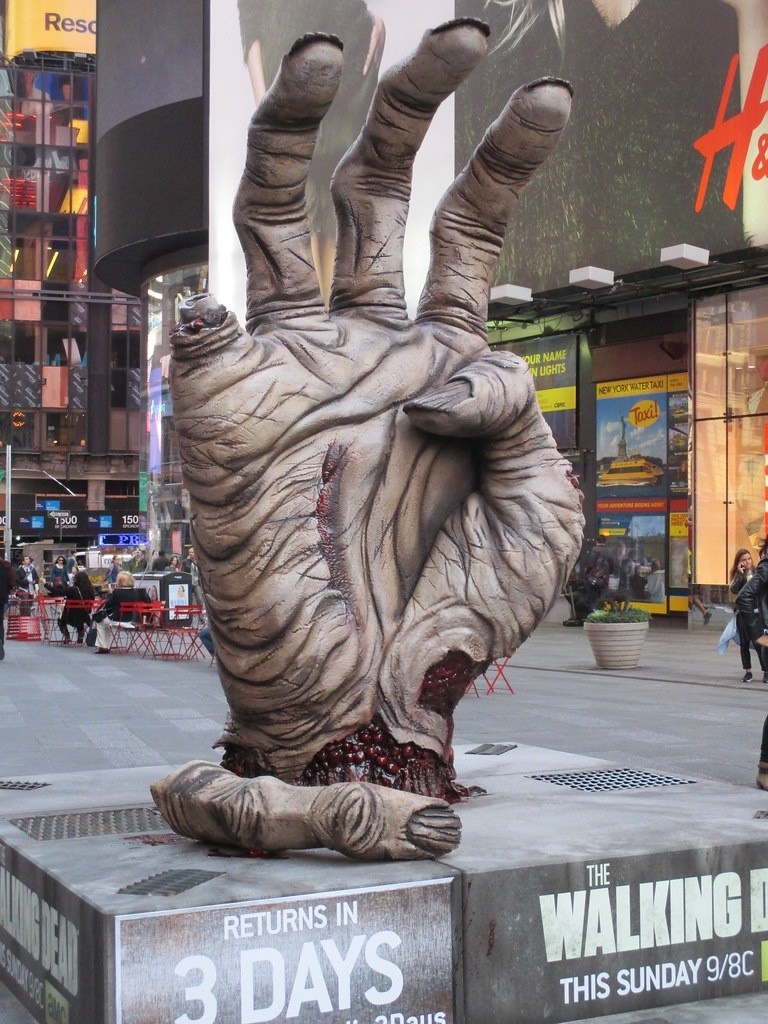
[763,672,768,683]
[741,672,753,683]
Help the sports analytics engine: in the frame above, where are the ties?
[25,568,29,573]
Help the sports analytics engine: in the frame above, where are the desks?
[9,599,38,640]
[40,603,67,646]
[149,607,194,662]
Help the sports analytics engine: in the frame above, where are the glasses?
[58,559,64,562]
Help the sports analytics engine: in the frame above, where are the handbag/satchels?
[587,546,611,589]
[53,576,63,587]
[85,619,98,647]
[84,614,92,627]
[92,608,113,623]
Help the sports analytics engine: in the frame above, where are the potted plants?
[580,589,651,672]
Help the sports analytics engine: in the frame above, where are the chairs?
[6,596,208,662]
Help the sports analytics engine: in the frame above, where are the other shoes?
[703,612,712,625]
[615,576,620,579]
[77,635,84,643]
[64,636,70,644]
[93,647,110,654]
[0,639,5,661]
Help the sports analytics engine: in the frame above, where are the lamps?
[660,243,768,275]
[22,48,38,69]
[568,266,704,300]
[490,284,618,311]
[74,53,87,70]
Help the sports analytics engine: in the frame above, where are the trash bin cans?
[128,568,194,628]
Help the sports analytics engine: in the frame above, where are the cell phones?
[741,564,746,573]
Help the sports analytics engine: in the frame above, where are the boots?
[756,762,768,791]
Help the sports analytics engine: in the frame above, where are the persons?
[687,546,712,626]
[587,534,613,614]
[718,528,767,684]
[0,548,222,659]
[756,704,768,794]
